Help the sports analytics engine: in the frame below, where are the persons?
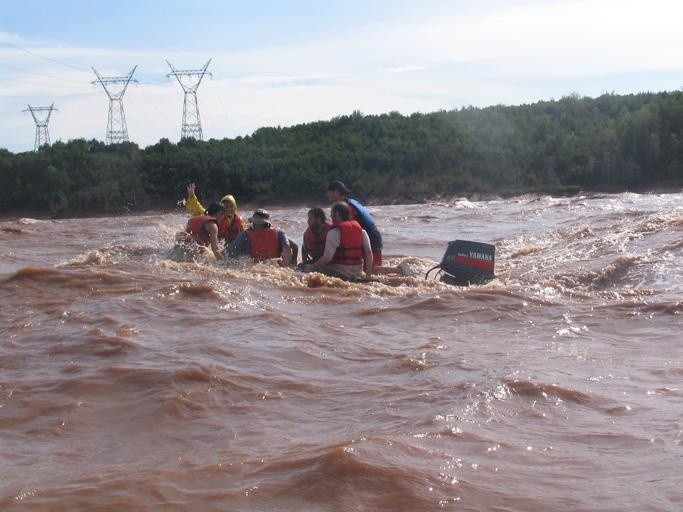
[186,202,226,260]
[326,180,382,266]
[298,207,333,271]
[225,209,292,267]
[301,201,373,283]
[184,182,246,241]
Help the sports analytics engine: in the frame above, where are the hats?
[327,180,353,196]
[247,208,272,225]
